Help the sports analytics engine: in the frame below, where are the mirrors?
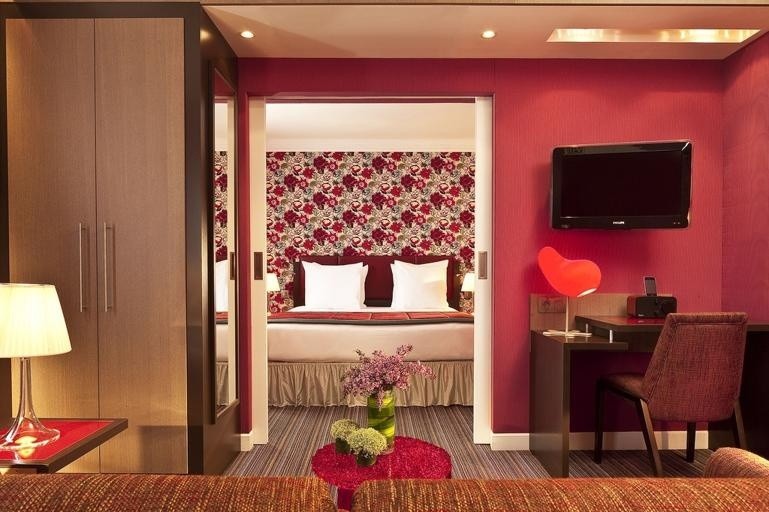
[205,62,242,427]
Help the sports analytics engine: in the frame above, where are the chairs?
[593,310,750,477]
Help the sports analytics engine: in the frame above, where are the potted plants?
[345,427,388,467]
[329,417,360,454]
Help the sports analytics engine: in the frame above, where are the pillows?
[389,259,450,311]
[214,258,229,313]
[301,259,369,311]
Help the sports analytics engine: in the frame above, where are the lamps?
[460,271,474,294]
[267,271,281,293]
[0,283,72,444]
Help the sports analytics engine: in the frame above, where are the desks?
[0,410,128,475]
[527,314,769,478]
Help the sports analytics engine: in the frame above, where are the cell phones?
[645,277,656,296]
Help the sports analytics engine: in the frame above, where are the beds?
[215,310,229,406]
[266,254,474,409]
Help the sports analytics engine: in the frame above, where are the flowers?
[339,343,439,413]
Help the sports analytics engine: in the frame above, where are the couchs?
[0,448,769,512]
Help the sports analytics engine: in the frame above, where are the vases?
[366,387,397,456]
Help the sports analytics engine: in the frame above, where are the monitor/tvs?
[551,141,691,228]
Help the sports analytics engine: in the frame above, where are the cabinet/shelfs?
[0,0,195,474]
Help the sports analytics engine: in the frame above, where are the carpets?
[307,434,453,486]
